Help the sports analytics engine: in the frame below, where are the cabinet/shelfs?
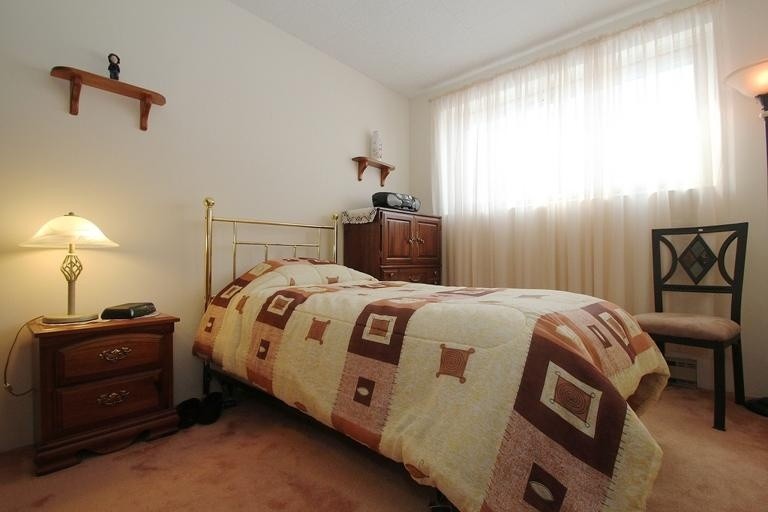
[343,207,443,285]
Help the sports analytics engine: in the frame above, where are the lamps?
[18,212,121,324]
[724,60,768,173]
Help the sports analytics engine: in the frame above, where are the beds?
[194,198,669,512]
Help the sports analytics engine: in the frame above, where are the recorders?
[372,192,421,212]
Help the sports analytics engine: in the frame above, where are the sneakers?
[175,392,224,428]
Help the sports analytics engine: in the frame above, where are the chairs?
[632,222,749,431]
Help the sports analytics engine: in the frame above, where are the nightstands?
[27,310,181,477]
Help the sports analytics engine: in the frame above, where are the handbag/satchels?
[101,302,156,319]
